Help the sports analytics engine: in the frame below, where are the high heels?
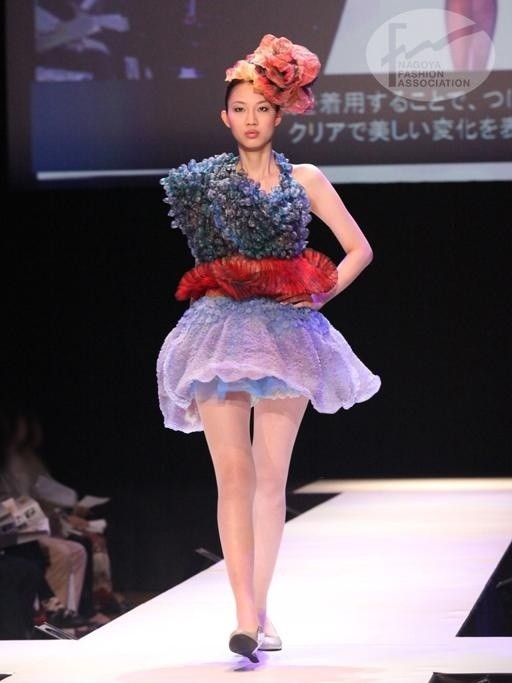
[229,626,281,663]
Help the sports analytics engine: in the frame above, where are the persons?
[0,410,131,640]
[444,0,499,72]
[155,32,383,665]
